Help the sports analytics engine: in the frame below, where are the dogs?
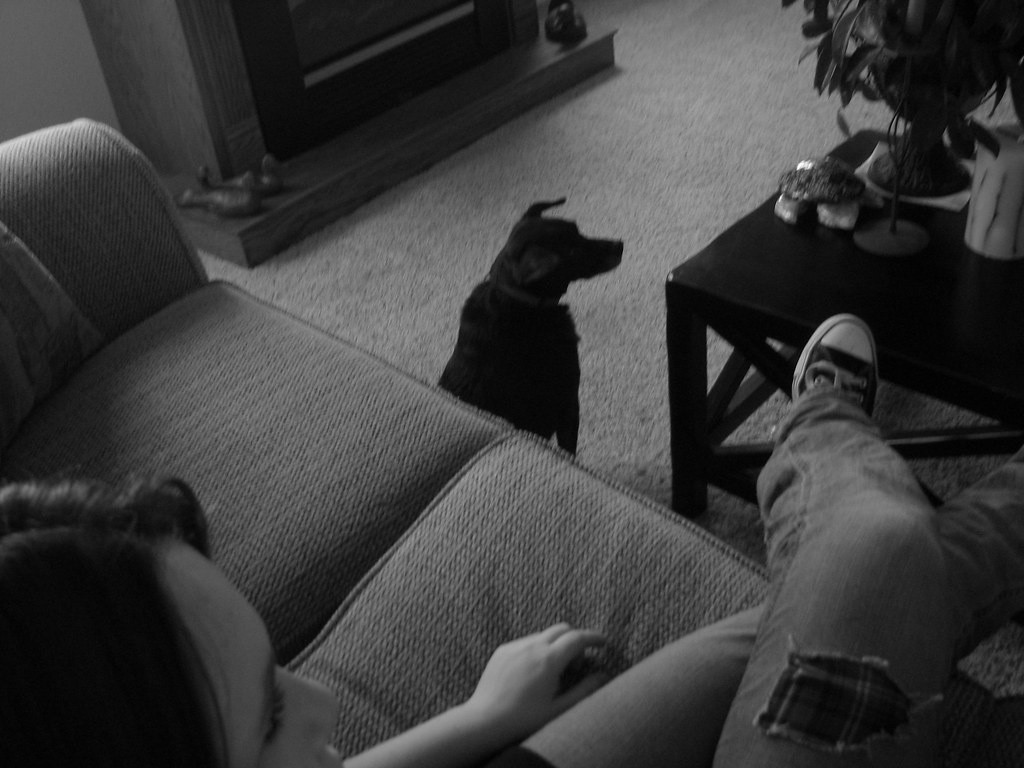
[435,196,625,456]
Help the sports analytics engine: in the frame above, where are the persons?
[0,310,1023,768]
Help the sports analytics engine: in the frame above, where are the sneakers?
[791,313,878,423]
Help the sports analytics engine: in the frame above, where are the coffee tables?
[663,131,1024,633]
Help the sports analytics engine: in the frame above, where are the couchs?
[0,119,1024,768]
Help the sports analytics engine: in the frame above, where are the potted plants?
[778,0,1023,198]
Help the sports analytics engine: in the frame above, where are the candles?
[963,123,1024,260]
